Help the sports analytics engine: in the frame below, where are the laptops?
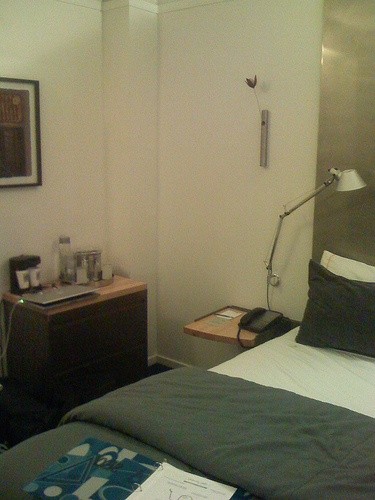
[23,285,100,310]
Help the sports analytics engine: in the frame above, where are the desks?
[184,306,300,347]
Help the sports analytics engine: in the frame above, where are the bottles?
[58,235,75,280]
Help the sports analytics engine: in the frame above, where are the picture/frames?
[0,77,43,188]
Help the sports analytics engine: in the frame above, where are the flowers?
[244,74,262,119]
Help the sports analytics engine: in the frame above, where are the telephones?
[238,306,284,332]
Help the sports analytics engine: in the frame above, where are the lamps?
[265,168,367,286]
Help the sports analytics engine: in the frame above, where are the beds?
[0,250,375,500]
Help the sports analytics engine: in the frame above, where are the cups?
[30,268,40,287]
[15,270,30,290]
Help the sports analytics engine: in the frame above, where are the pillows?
[295,250,375,357]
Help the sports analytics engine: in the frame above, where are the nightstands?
[2,275,148,410]
